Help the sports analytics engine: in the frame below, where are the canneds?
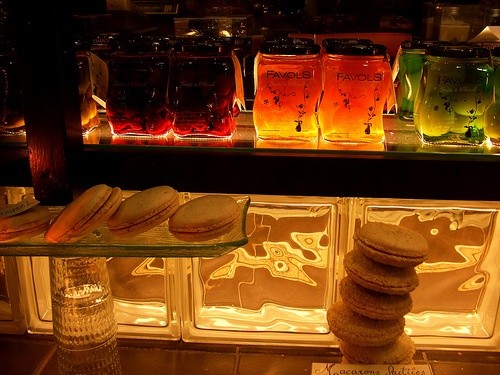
[413,45,492,145]
[0,39,94,145]
[106,34,173,140]
[491,47,500,146]
[400,39,434,122]
[171,35,234,139]
[253,41,323,141]
[317,44,389,144]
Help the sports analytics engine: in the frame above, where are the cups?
[48,257,118,352]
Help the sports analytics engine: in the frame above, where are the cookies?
[106,185,179,238]
[169,194,243,242]
[44,183,123,244]
[327,221,430,365]
[0,197,52,244]
